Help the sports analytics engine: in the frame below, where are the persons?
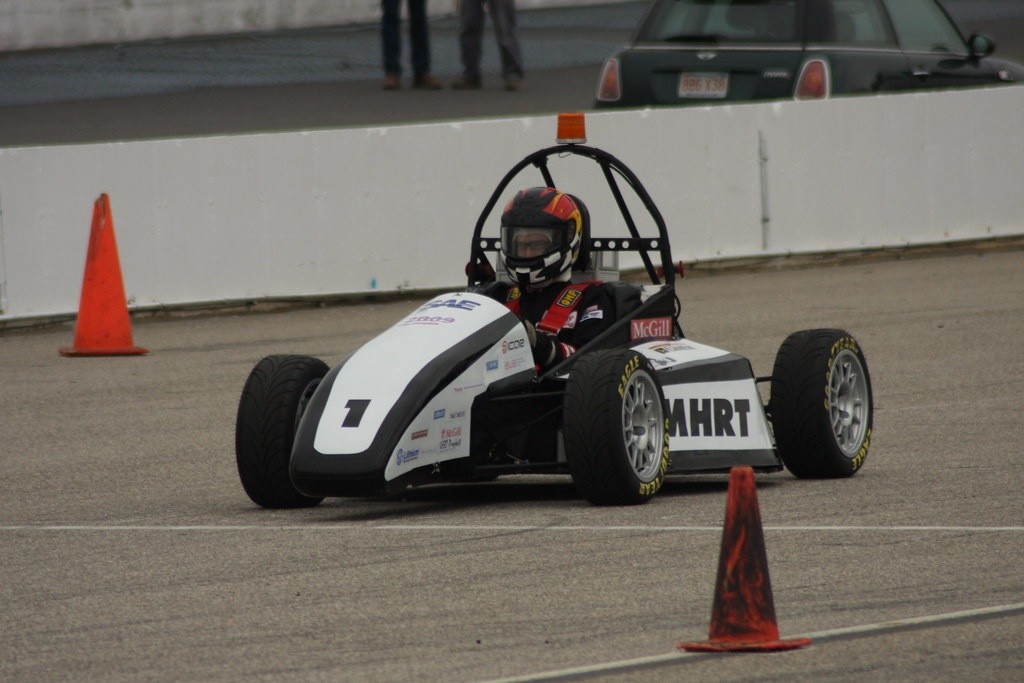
[448,0,524,92]
[379,0,443,90]
[463,186,618,383]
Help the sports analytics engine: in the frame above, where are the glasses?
[512,238,550,251]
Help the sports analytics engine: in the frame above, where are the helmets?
[499,187,583,290]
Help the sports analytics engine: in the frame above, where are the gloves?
[520,320,561,365]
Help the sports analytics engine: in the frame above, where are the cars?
[592,0,1024,109]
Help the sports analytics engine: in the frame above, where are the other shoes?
[451,75,482,91]
[412,75,435,90]
[380,71,399,90]
[504,71,521,89]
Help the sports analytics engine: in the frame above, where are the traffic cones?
[677,466,812,653]
[58,194,150,356]
